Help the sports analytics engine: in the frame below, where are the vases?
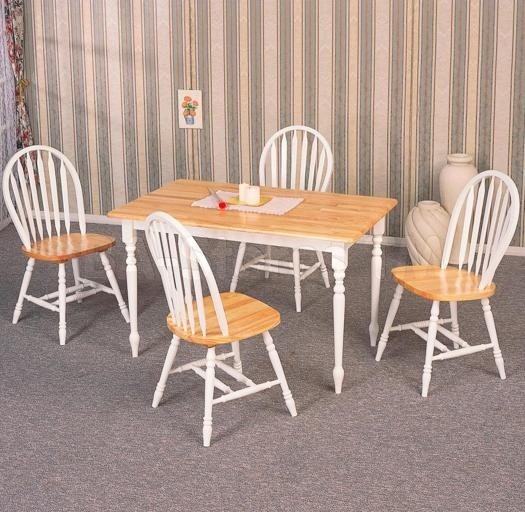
[404,200,450,266]
[438,153,481,266]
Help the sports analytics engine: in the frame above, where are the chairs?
[143,210,298,449]
[373,169,521,398]
[0,144,130,345]
[229,124,333,312]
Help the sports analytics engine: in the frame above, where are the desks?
[106,179,399,395]
